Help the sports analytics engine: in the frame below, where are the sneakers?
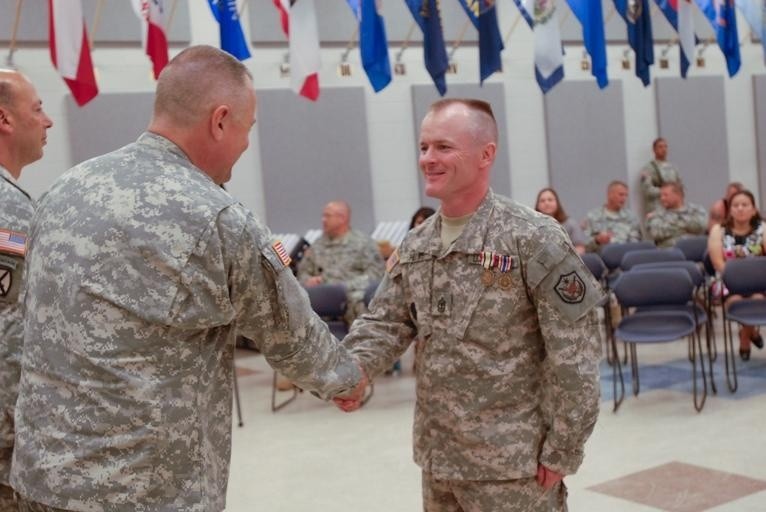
[749,327,763,349]
[738,330,750,361]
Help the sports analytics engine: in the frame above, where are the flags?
[460,1,506,85]
[208,1,256,62]
[569,0,610,90]
[736,1,766,60]
[46,2,99,106]
[271,1,329,101]
[695,1,741,79]
[612,0,654,87]
[656,1,701,81]
[344,1,392,93]
[513,1,566,95]
[403,0,451,96]
[131,2,170,81]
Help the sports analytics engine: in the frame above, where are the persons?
[274,199,384,392]
[408,137,766,361]
[11,43,367,511]
[336,97,609,512]
[0,65,54,512]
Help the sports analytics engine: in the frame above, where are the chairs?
[270,282,377,417]
[578,233,765,415]
